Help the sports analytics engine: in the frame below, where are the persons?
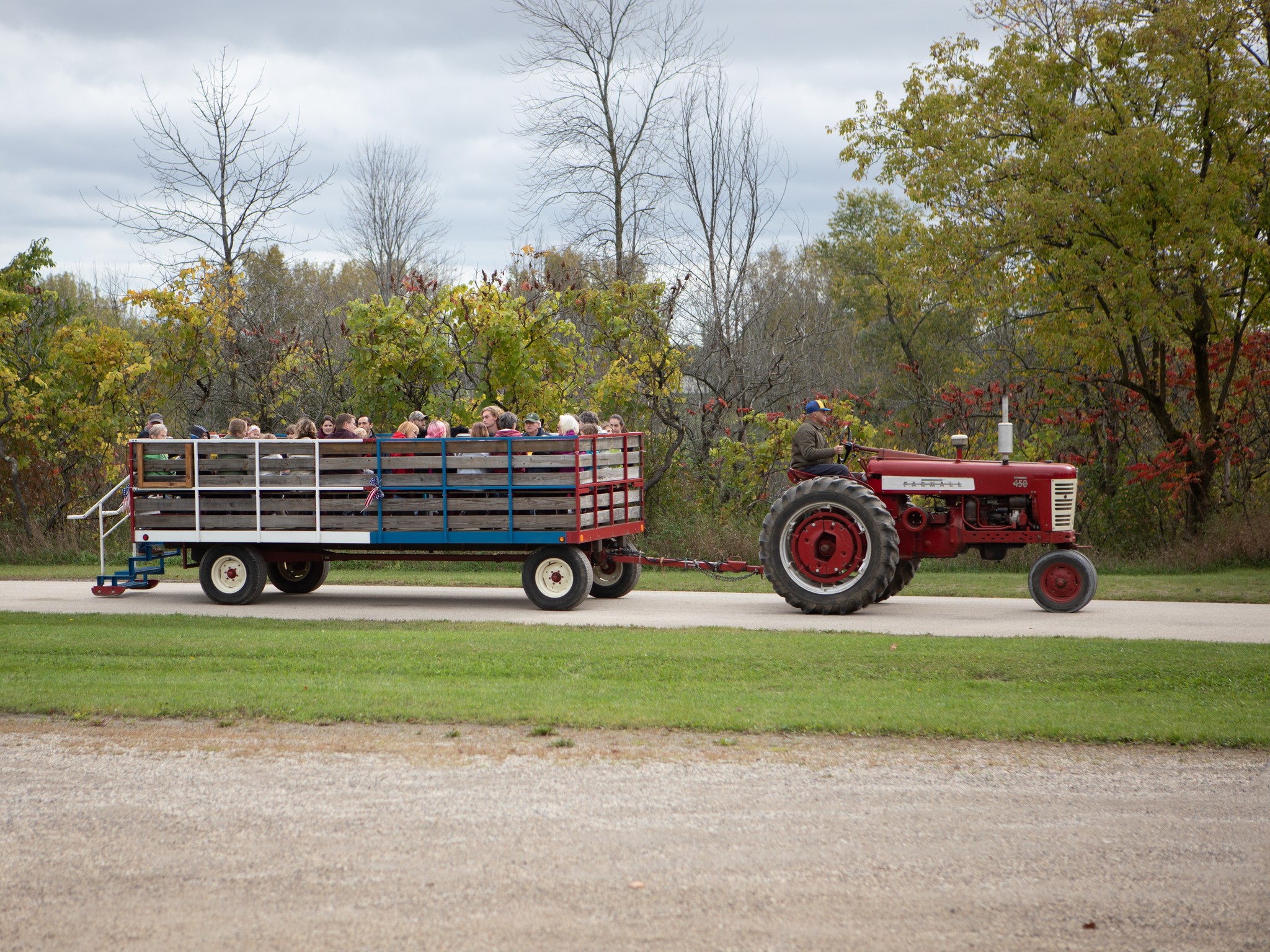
[137,406,627,530]
[791,400,850,477]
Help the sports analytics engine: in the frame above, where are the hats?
[408,411,429,423]
[805,400,831,414]
[148,413,163,423]
[523,413,540,423]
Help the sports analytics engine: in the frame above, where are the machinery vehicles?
[760,393,1099,616]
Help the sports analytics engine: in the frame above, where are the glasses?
[343,422,356,426]
[201,434,209,438]
[556,425,560,429]
[247,423,251,428]
